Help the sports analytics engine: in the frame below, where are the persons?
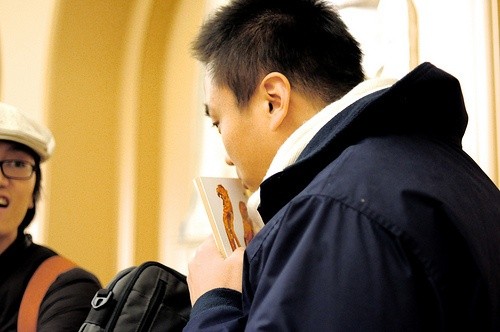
[180,1,500,331]
[0,102,102,332]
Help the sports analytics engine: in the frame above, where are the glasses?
[0,159,37,180]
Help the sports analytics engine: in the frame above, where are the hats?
[0,103,55,164]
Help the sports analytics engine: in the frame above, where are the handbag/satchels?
[77,261,192,332]
